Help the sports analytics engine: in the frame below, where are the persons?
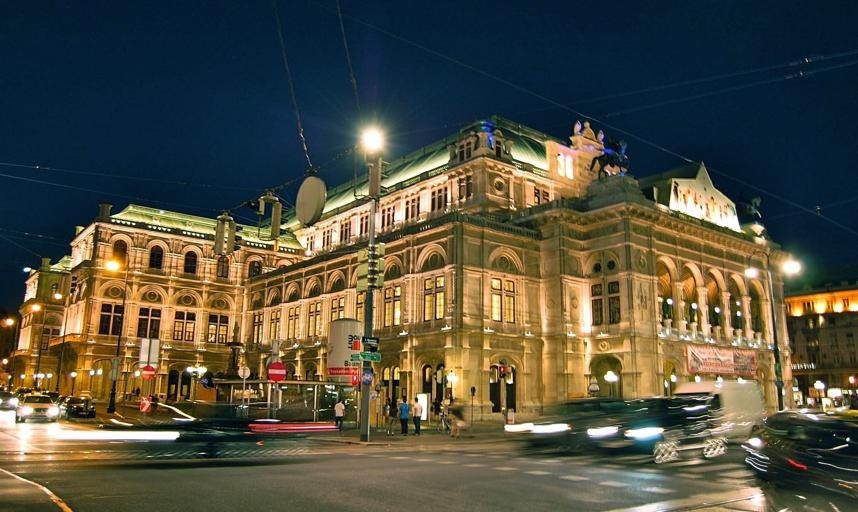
[412,397,423,436]
[390,399,398,424]
[398,397,410,434]
[850,390,858,409]
[334,399,345,433]
[383,399,399,436]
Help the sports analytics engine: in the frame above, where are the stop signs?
[142,366,156,381]
[268,363,287,382]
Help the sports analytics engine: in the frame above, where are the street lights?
[357,128,384,442]
[603,370,623,398]
[32,304,47,389]
[744,247,801,411]
[186,366,207,402]
[447,372,457,401]
[55,292,71,393]
[106,253,130,414]
[6,318,20,392]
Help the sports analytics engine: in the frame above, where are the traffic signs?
[361,336,380,346]
[358,352,382,363]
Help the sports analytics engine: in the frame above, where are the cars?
[0,386,96,422]
